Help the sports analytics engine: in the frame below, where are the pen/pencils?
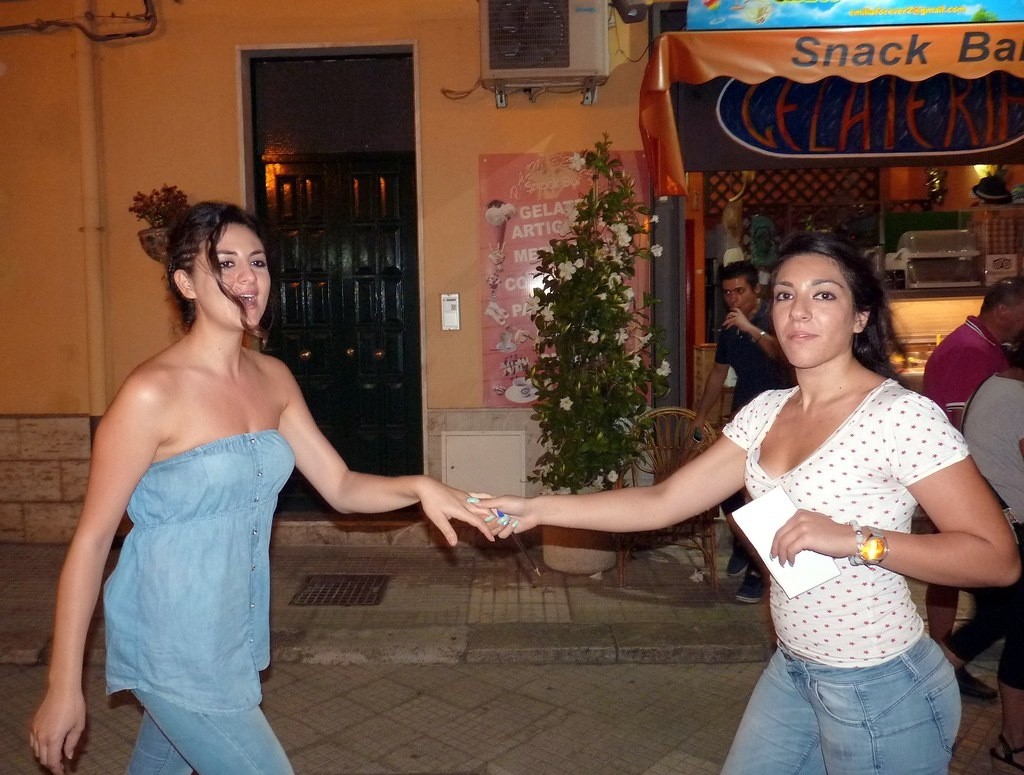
[496,508,540,578]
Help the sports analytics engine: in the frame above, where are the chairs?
[612,404,722,596]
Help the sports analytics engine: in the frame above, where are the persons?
[472,237,1021,775]
[691,261,796,602]
[30,202,501,775]
[921,278,1024,775]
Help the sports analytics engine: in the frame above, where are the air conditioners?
[478,0,611,91]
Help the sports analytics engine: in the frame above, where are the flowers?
[506,128,673,498]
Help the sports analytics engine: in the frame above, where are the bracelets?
[752,331,766,342]
[847,520,863,566]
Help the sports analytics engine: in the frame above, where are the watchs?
[862,525,888,565]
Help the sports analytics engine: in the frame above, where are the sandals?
[989,734,1024,775]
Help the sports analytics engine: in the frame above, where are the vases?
[541,487,618,577]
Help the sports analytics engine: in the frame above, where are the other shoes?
[726,553,749,577]
[955,666,998,699]
[736,574,770,603]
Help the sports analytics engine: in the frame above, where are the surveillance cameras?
[612,0,647,23]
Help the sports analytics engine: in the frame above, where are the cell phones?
[693,427,702,443]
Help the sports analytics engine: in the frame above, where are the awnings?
[638,21,1024,198]
